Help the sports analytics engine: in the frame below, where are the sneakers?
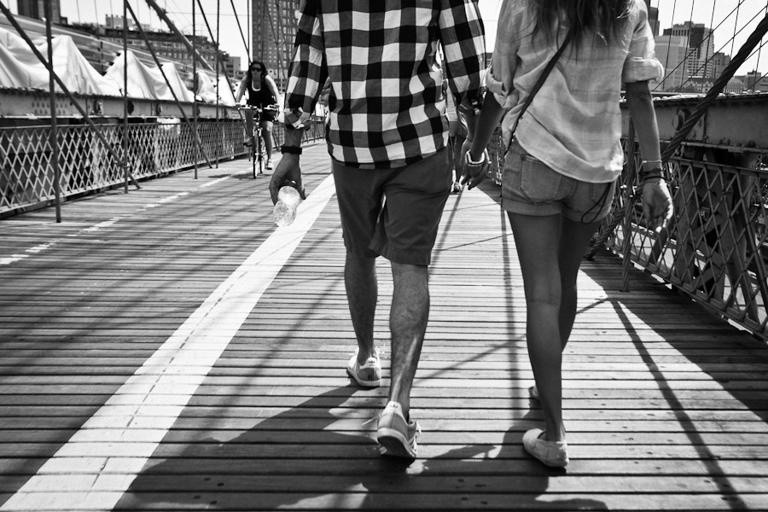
[522,427,569,467]
[529,385,542,400]
[347,354,382,387]
[376,401,419,457]
[242,138,251,145]
[454,181,460,191]
[266,161,273,169]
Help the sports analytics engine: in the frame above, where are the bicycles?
[232,106,278,179]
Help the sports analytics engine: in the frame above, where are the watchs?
[464,149,485,167]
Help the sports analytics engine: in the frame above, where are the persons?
[268,0,490,460]
[442,75,470,192]
[463,0,675,468]
[235,60,280,170]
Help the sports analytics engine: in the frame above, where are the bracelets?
[280,145,302,154]
[640,168,665,176]
[644,176,666,181]
[640,160,663,165]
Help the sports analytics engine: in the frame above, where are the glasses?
[250,66,260,72]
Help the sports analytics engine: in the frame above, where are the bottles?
[271,180,302,229]
